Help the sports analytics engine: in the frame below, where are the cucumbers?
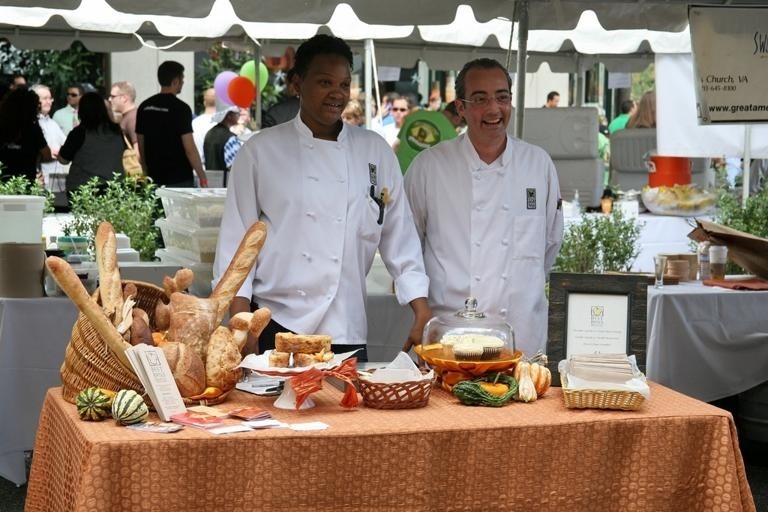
[455,370,517,407]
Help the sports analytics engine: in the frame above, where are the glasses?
[393,108,405,111]
[67,92,78,97]
[460,95,511,106]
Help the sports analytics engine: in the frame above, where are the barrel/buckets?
[0,194,46,245]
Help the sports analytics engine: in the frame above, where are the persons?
[0,73,140,213]
[626,91,657,128]
[542,92,560,108]
[211,33,432,363]
[342,72,468,177]
[135,61,210,246]
[262,66,301,128]
[392,58,564,365]
[203,105,256,170]
[608,100,637,133]
[191,88,217,190]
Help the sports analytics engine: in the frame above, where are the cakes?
[440,332,505,362]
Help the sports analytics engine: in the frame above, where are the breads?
[44,220,271,400]
[269,331,335,370]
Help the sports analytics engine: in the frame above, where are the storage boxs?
[153,189,227,299]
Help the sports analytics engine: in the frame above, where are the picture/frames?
[549,272,647,388]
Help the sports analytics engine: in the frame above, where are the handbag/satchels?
[50,191,69,207]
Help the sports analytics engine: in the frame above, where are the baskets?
[559,371,648,411]
[60,279,235,410]
[357,366,437,409]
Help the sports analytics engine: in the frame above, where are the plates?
[724,275,754,281]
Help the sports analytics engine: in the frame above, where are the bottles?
[699,240,710,280]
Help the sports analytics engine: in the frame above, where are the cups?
[654,257,666,285]
[710,246,727,280]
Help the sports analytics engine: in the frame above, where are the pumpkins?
[512,361,551,400]
[76,384,149,425]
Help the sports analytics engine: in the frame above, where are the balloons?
[214,59,270,115]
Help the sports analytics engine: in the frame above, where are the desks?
[645,273,768,398]
[0,297,92,487]
[562,210,722,274]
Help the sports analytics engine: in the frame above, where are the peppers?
[479,371,508,396]
